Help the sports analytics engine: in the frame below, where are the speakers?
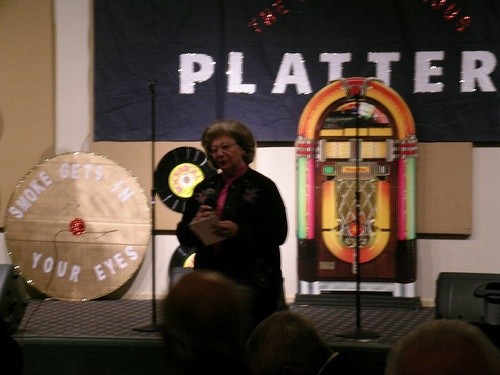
[436,272,500,349]
[0,264,28,334]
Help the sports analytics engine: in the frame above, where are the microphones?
[202,188,218,212]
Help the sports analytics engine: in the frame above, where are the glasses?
[210,143,238,153]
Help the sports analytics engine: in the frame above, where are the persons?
[176,120,288,374]
[163,271,500,375]
